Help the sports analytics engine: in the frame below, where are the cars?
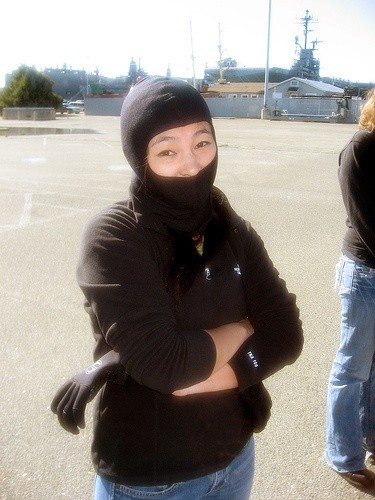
[63,99,84,114]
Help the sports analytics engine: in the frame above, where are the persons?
[77,76,304,500]
[323,85,375,492]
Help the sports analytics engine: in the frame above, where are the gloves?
[50,349,123,436]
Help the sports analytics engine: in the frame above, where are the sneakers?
[340,469,375,489]
